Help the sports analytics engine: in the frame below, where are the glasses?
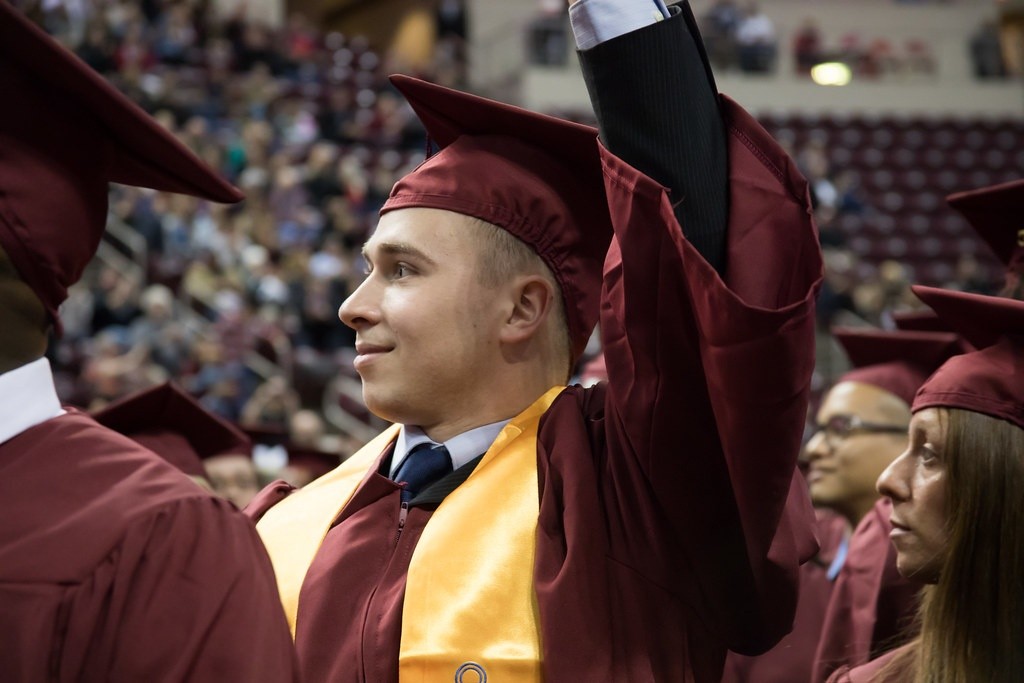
[802,414,907,440]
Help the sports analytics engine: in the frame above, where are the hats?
[946,177,1024,285]
[87,379,253,478]
[376,69,615,378]
[826,325,965,409]
[892,307,980,355]
[240,428,283,459]
[281,435,343,471]
[0,0,245,317]
[902,285,1024,433]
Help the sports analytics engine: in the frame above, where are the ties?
[392,439,454,505]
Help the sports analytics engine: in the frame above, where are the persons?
[0,0,301,683]
[0,0,919,451]
[84,381,343,525]
[722,175,1023,683]
[238,0,823,683]
